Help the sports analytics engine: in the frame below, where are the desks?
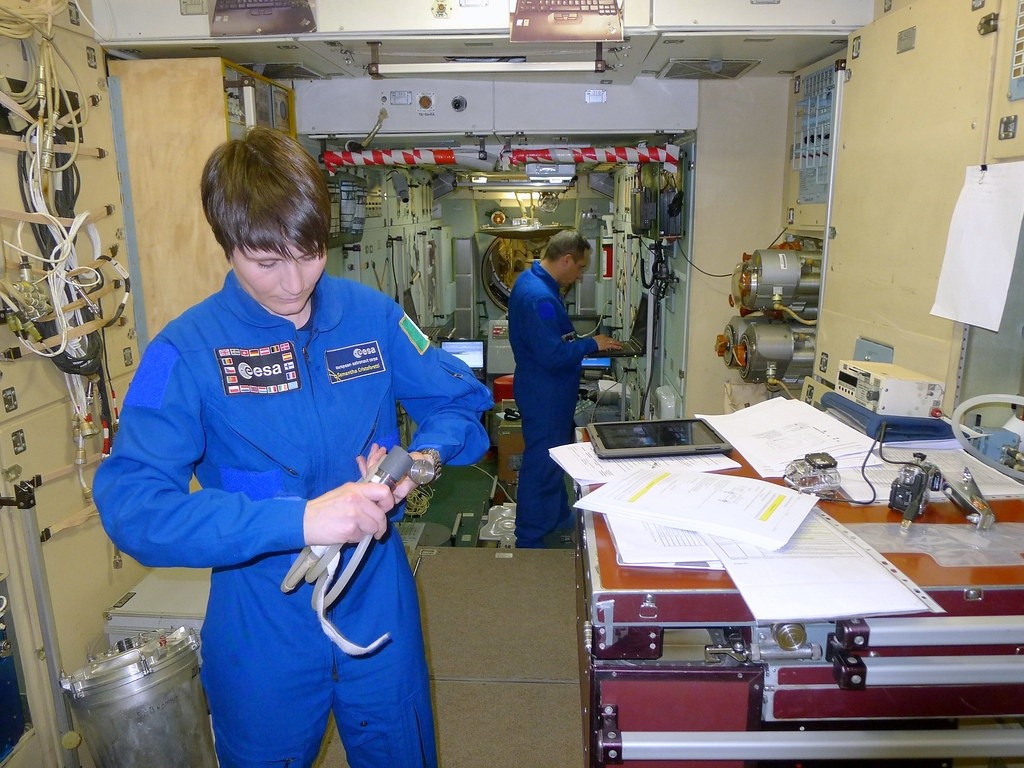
[576,425,1024,768]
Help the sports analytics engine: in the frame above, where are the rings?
[609,341,612,344]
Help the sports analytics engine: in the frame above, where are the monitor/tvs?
[581,356,611,369]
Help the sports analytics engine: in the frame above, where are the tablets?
[585,418,733,459]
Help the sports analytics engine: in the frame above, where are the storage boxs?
[498,418,526,484]
[102,568,213,668]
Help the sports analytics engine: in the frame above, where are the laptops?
[440,339,486,386]
[589,292,656,357]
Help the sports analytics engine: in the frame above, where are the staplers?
[937,465,995,531]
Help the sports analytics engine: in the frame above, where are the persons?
[92,127,495,768]
[508,230,624,549]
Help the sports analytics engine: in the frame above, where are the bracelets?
[422,448,441,483]
